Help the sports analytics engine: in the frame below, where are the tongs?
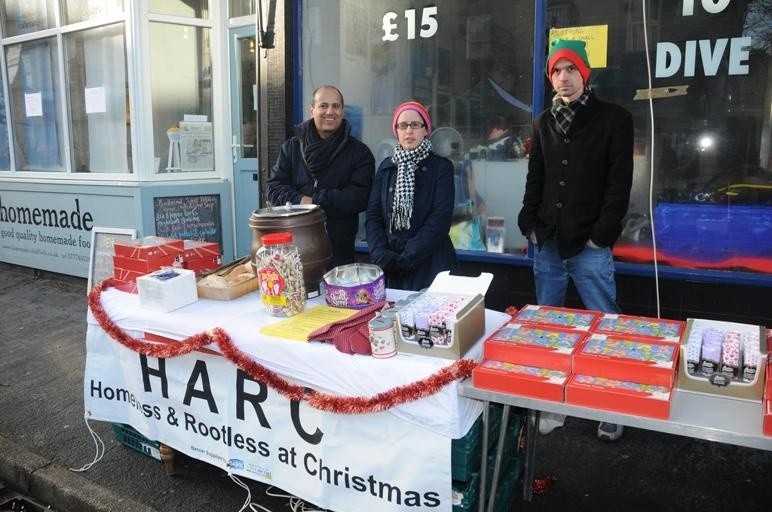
[201,256,250,278]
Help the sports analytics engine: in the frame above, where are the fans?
[429,126,464,158]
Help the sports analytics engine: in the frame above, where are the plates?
[195,275,259,301]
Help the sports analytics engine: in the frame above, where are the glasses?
[395,121,425,129]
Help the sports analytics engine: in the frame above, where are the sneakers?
[598,421,624,441]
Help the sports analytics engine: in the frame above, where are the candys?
[257,245,305,317]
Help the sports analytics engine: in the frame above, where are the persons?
[517,39,633,320]
[264,84,374,269]
[366,102,465,294]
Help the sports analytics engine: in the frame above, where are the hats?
[545,38,591,83]
[392,101,431,139]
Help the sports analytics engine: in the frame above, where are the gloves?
[384,256,402,273]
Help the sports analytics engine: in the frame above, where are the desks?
[457,375,772,512]
[84,285,452,476]
